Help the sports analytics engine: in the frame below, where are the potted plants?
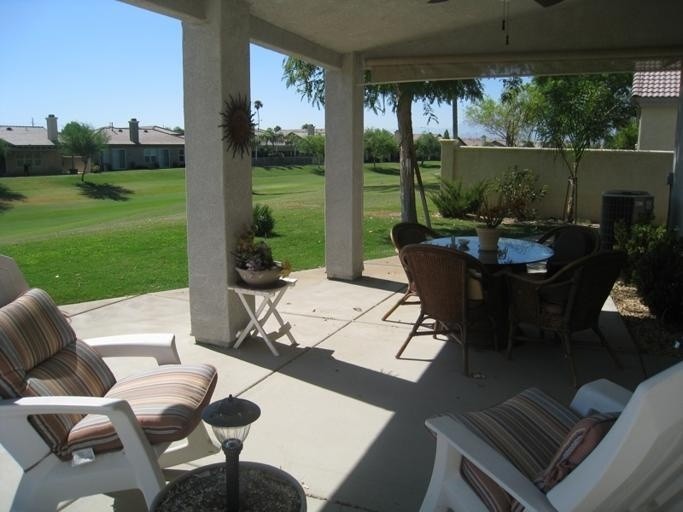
[474,168,548,254]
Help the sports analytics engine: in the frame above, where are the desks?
[422,233,554,363]
[150,457,308,512]
[228,278,300,356]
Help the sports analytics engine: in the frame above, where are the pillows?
[508,412,621,512]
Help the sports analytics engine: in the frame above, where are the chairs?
[387,220,494,381]
[502,218,629,390]
[416,361,683,511]
[0,286,218,510]
